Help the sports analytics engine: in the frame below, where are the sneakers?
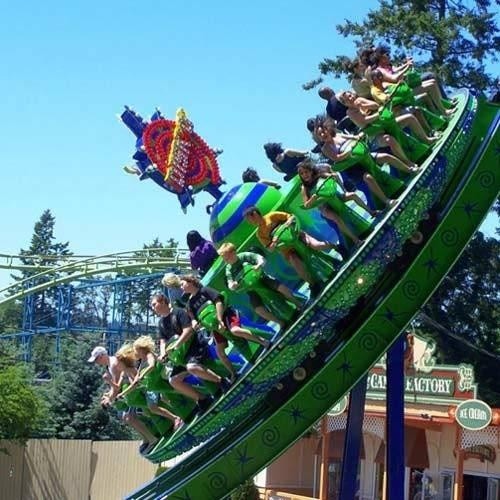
[197,395,214,417]
[372,199,397,218]
[310,283,321,298]
[405,160,422,177]
[220,369,237,395]
[279,322,285,336]
[174,417,182,430]
[295,304,304,317]
[444,98,458,115]
[264,340,271,351]
[428,129,442,145]
[336,240,365,260]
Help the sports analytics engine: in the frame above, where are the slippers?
[140,439,162,454]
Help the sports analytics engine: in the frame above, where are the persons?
[86,46,461,454]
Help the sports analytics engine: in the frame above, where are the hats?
[88,347,106,363]
